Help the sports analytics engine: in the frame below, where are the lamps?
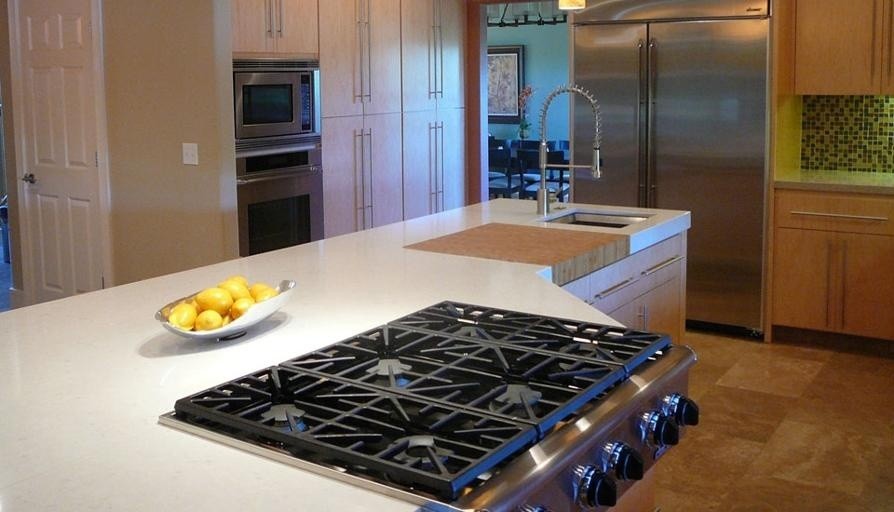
[558,0,586,9]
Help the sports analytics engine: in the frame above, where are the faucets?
[536,85,602,215]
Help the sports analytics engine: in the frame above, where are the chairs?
[488,136,570,202]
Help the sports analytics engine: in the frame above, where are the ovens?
[231,57,325,258]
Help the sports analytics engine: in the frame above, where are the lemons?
[167,275,280,333]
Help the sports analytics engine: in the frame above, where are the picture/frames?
[487,44,525,124]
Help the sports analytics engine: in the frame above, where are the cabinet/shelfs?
[772,1,894,96]
[230,1,319,54]
[318,1,404,238]
[401,1,470,221]
[559,230,688,346]
[763,180,894,344]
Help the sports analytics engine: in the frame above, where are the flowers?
[519,87,531,130]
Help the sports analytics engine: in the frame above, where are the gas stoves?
[170,298,703,512]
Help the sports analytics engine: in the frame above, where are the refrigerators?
[565,0,770,338]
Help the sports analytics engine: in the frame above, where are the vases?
[520,130,530,140]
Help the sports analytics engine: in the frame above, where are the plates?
[153,279,297,341]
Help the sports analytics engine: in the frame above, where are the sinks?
[546,211,655,229]
